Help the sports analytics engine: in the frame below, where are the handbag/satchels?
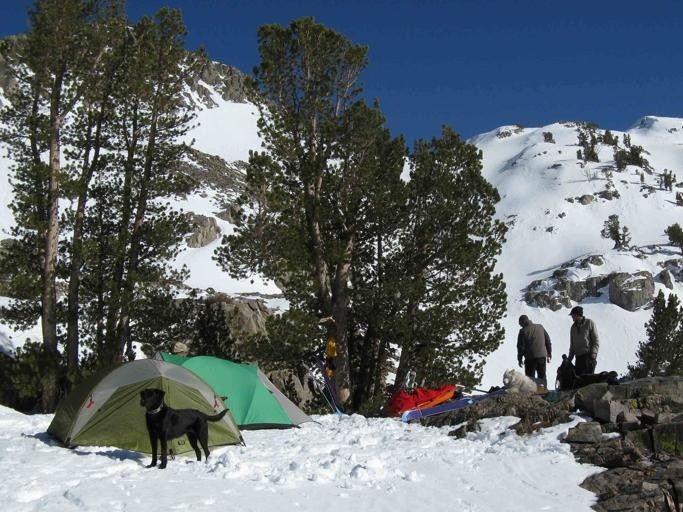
[582,371,620,386]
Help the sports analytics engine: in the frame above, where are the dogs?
[139,388,230,470]
[503,368,538,395]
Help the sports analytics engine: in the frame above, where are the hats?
[569,306,583,315]
[519,315,529,323]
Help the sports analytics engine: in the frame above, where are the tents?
[153,350,322,429]
[47,359,247,459]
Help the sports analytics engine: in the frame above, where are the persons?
[569,306,599,374]
[518,315,552,390]
